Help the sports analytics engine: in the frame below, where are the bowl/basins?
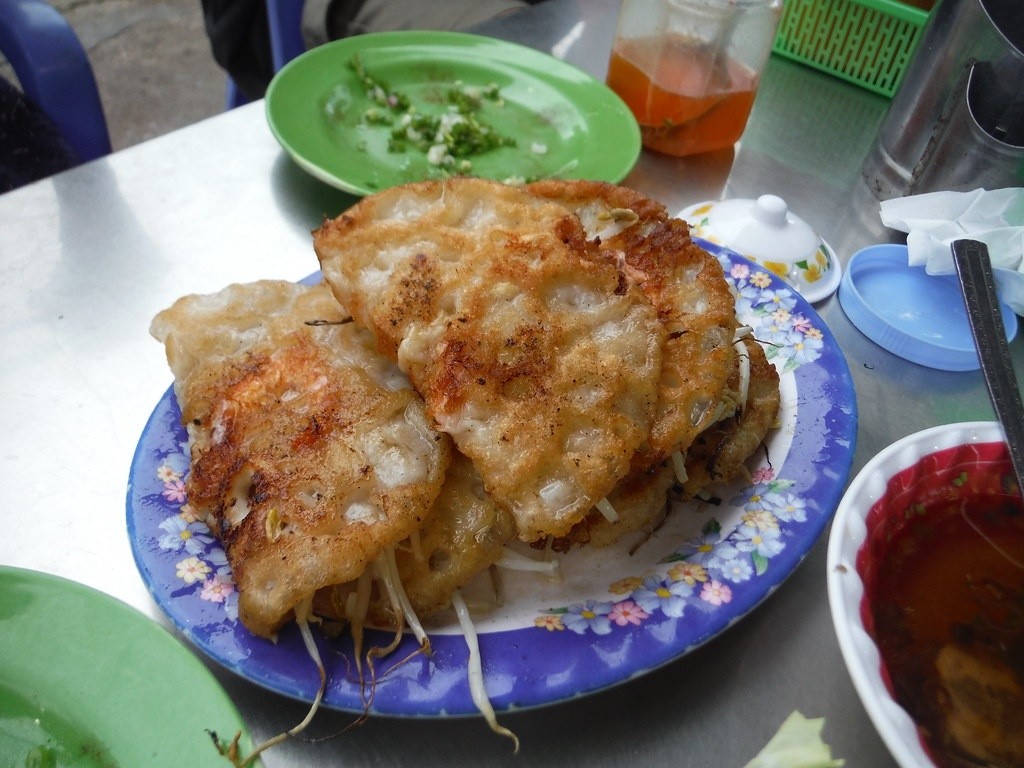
[825,421,1024,768]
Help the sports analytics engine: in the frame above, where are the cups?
[604,0,785,157]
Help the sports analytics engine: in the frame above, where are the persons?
[201,0,375,106]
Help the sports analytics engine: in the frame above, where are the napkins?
[879,186,1024,317]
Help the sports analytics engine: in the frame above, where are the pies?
[145,176,783,629]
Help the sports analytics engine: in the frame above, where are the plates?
[124,234,859,720]
[265,29,643,197]
[0,563,266,768]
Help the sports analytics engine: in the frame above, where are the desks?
[0,0,1024,768]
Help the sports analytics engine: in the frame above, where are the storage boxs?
[771,0,941,99]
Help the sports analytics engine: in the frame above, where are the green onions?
[343,57,519,176]
